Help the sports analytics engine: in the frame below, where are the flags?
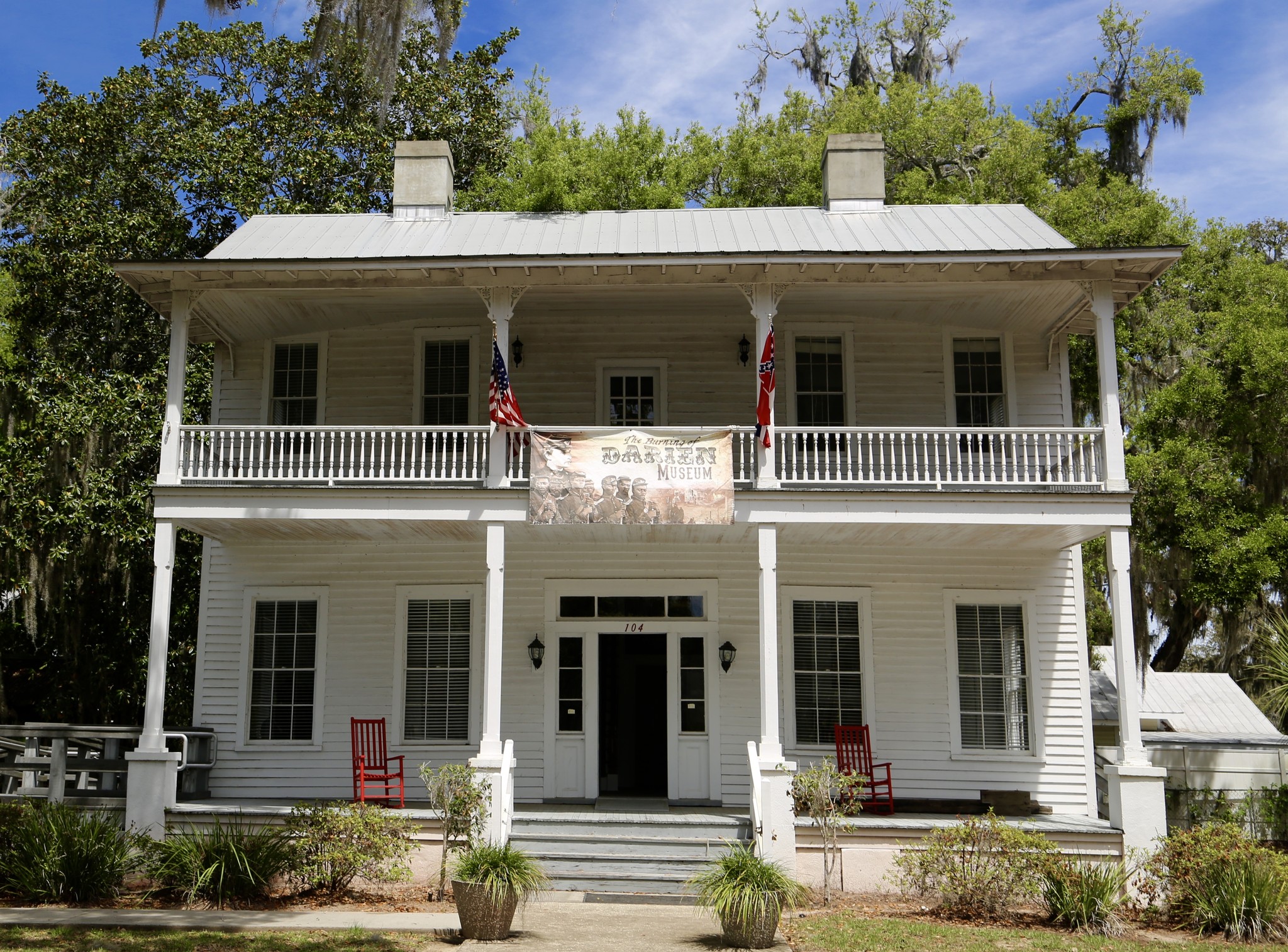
[488,332,534,464]
[753,323,777,447]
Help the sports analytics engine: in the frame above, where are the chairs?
[350,716,406,809]
[835,722,895,817]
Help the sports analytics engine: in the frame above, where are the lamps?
[737,333,751,367]
[511,335,524,368]
[527,632,546,672]
[718,641,737,674]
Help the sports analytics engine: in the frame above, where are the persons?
[529,434,696,524]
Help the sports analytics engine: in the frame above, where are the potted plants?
[677,837,821,949]
[445,823,556,943]
